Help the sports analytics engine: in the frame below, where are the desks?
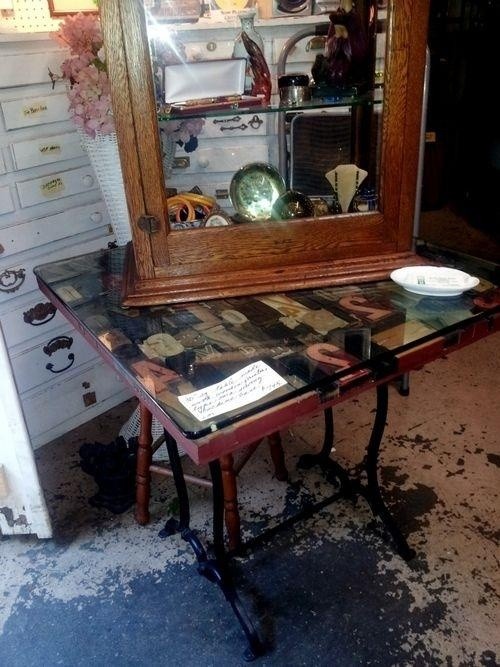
[34,244,500,659]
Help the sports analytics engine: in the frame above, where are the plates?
[391,265,482,296]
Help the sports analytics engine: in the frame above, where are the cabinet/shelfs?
[0,13,388,543]
[98,1,433,306]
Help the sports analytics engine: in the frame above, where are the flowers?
[52,15,204,141]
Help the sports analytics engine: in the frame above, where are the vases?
[80,130,180,247]
